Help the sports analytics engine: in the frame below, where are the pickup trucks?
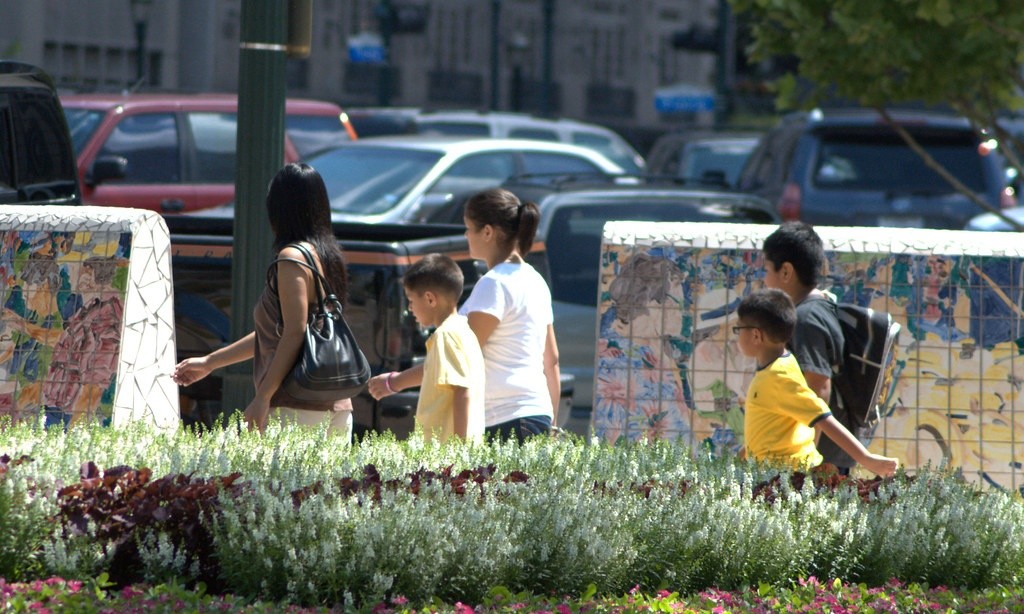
[0,58,556,442]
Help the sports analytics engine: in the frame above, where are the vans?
[55,88,365,216]
[407,110,647,181]
[731,102,1020,231]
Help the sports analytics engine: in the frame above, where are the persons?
[167,163,370,460]
[365,187,563,469]
[398,253,486,458]
[293,243,354,453]
[733,287,901,491]
[762,215,900,490]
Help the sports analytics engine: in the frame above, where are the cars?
[168,132,632,234]
[531,188,784,436]
[645,131,764,185]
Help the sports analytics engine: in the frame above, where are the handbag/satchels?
[265,244,372,402]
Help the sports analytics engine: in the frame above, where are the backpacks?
[795,297,902,428]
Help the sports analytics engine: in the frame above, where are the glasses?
[732,326,759,334]
